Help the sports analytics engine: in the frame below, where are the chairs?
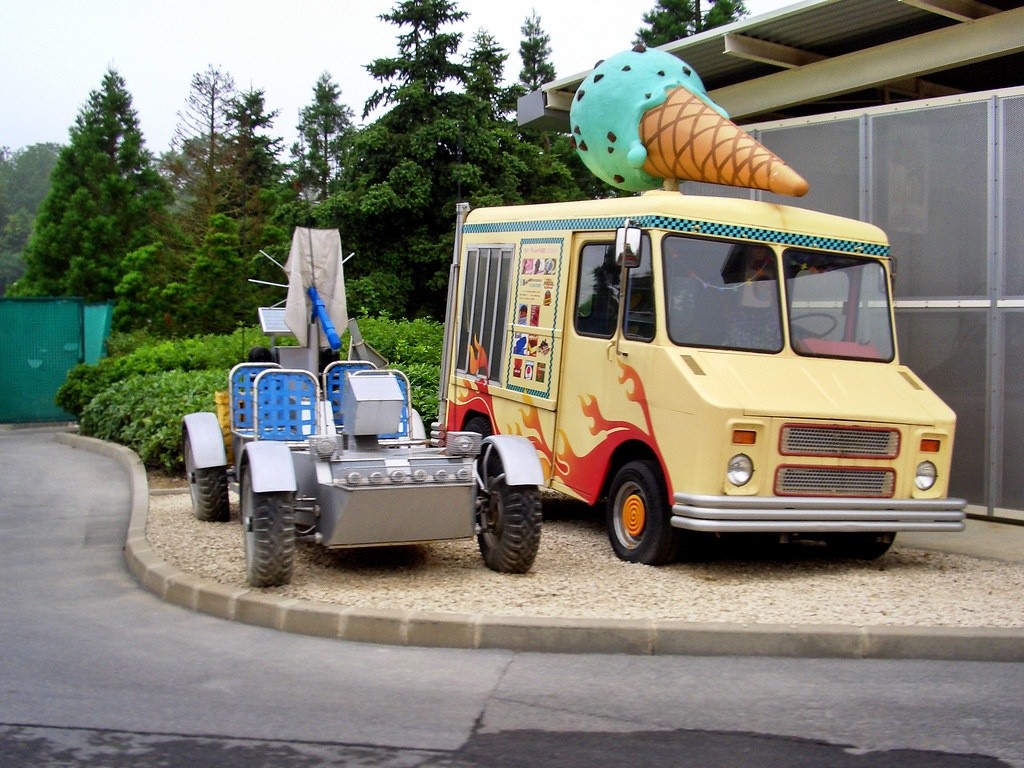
[322,359,378,434]
[252,370,322,449]
[352,369,414,445]
[228,362,284,439]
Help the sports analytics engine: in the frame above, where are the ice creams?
[517,258,555,328]
[570,42,808,196]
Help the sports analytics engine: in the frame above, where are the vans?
[430,189,968,567]
